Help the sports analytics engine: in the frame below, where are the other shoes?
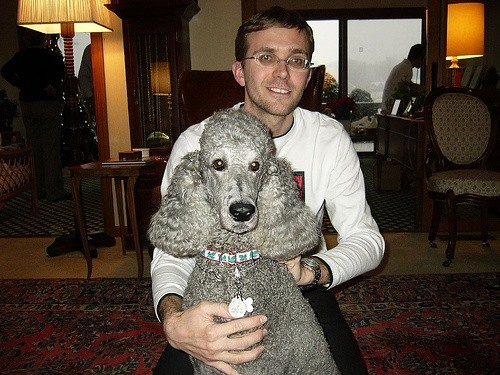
[46,191,58,201]
[31,190,46,200]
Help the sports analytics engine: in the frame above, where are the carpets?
[0,274,500,375]
[0,166,106,238]
[322,155,423,234]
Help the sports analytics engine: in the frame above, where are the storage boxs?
[373,160,402,192]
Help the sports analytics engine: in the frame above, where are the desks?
[68,156,165,281]
[376,112,433,229]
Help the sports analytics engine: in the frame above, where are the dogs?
[147,107,342,375]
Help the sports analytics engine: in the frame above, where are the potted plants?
[0,88,20,145]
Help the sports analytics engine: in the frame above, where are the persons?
[78,44,94,118]
[381,44,428,116]
[0,28,73,201]
[147,7,386,375]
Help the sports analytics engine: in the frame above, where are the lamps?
[445,3,485,68]
[16,0,115,167]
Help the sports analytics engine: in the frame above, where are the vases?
[338,119,352,132]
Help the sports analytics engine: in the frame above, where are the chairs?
[424,85,500,266]
[0,140,39,216]
[177,65,326,130]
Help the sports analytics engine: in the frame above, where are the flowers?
[326,96,358,120]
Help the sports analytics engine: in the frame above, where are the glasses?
[240,51,314,71]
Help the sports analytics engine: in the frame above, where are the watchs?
[298,257,321,291]
